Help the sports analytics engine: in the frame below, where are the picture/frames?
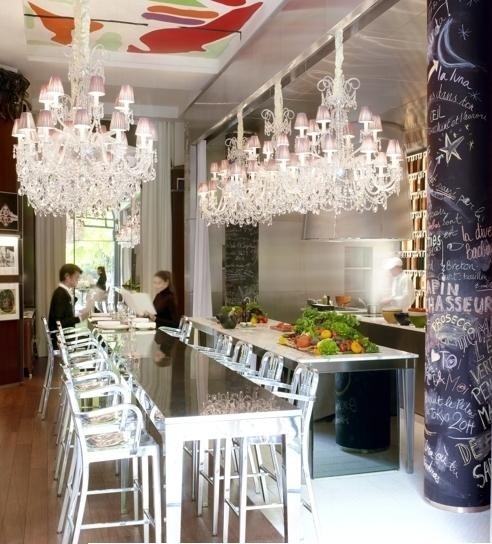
[0,189,21,232]
[0,229,21,278]
[0,280,22,323]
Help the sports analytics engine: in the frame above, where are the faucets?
[366,303,379,314]
[357,297,366,309]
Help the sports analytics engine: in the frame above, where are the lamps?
[8,1,158,219]
[68,203,141,249]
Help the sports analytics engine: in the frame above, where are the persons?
[378,257,415,313]
[136,270,179,330]
[48,263,91,350]
[95,265,107,291]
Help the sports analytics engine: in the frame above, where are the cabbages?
[317,339,339,355]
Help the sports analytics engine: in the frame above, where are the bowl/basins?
[409,312,427,328]
[394,313,410,325]
[336,296,352,307]
[383,310,402,323]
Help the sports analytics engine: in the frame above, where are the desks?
[184,313,420,479]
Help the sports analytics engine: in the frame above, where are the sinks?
[361,314,382,318]
[334,308,359,312]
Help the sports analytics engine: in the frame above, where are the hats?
[387,257,402,269]
